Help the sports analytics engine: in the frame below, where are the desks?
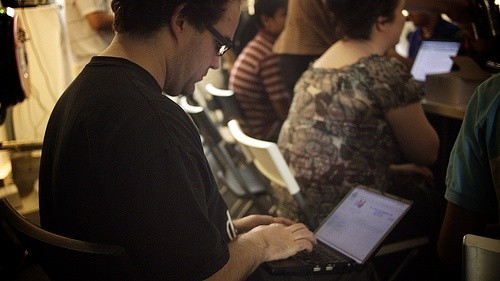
[420,93,468,121]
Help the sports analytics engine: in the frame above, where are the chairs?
[227,119,430,281]
[178,66,275,222]
[1,197,132,281]
[462,235,500,281]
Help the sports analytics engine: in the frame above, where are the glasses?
[183,3,234,56]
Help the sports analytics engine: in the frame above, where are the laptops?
[410,39,463,88]
[260,182,413,275]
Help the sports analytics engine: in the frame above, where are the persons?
[228,0,292,141]
[433,73,500,281]
[39,0,317,281]
[277,0,440,281]
[385,0,470,75]
